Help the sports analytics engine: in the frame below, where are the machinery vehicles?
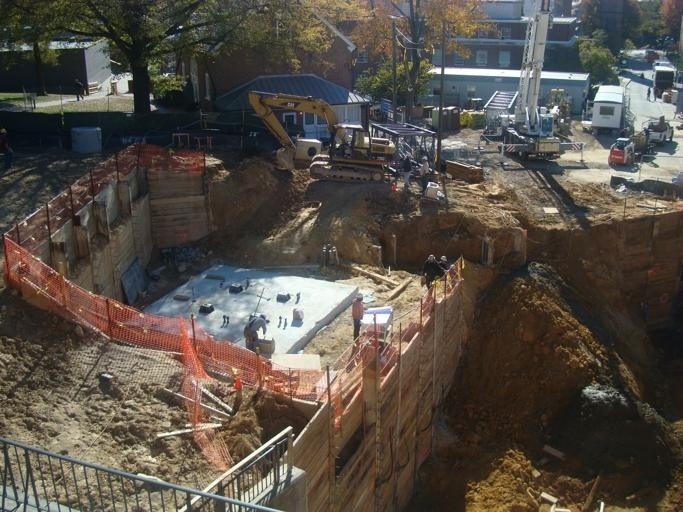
[474,0,584,166]
[248,91,398,184]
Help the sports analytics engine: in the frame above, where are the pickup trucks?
[648,121,673,146]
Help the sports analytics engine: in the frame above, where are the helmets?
[356,293,364,299]
[440,255,447,261]
[422,156,428,160]
[428,255,436,260]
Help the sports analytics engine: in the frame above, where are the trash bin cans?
[432,106,460,134]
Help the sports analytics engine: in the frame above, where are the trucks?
[652,66,674,98]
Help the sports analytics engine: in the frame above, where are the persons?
[352,293,364,341]
[653,87,658,101]
[233,377,243,399]
[419,155,430,194]
[75,78,84,101]
[423,254,448,290]
[243,312,269,343]
[449,263,457,288]
[619,127,631,137]
[402,156,412,183]
[438,255,450,282]
[647,87,651,101]
[643,126,649,147]
